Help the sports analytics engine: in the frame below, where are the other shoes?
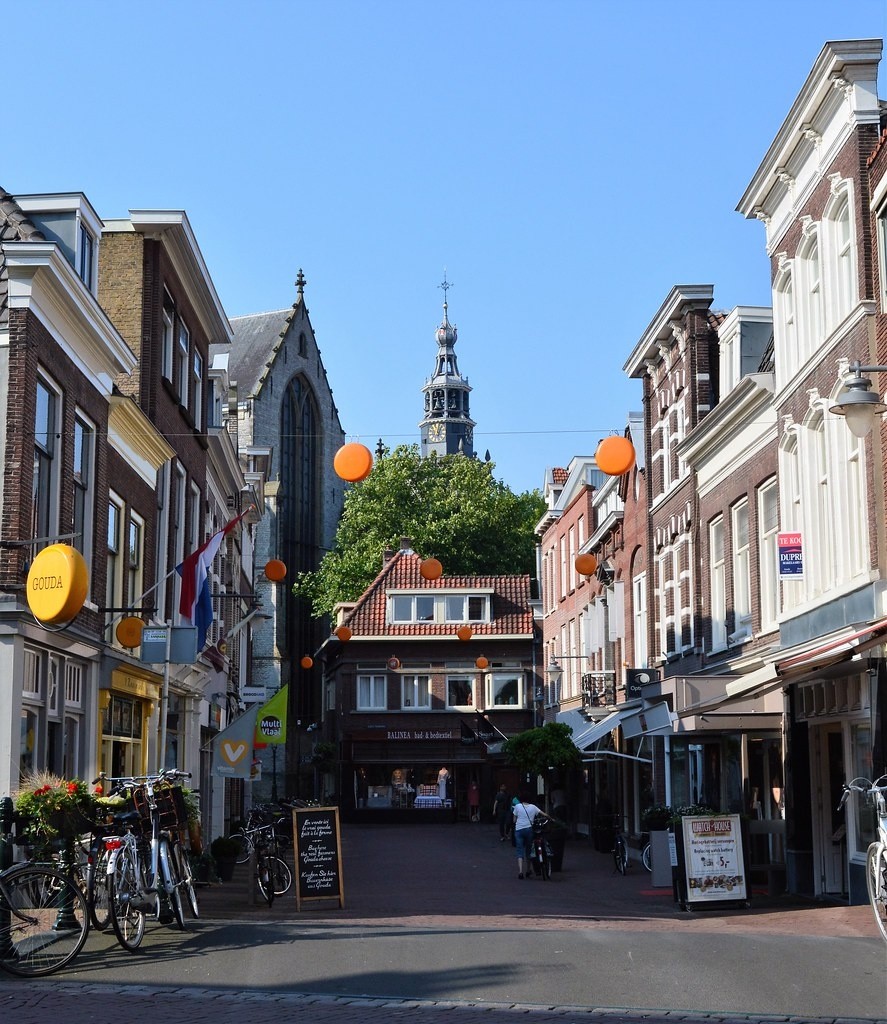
[519,873,523,880]
[526,872,529,877]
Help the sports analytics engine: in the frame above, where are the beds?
[414,786,442,808]
[368,786,392,808]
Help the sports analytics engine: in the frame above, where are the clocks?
[465,425,473,444]
[428,422,446,443]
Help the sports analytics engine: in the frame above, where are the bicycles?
[529,816,555,882]
[610,814,631,876]
[641,841,652,873]
[0,859,91,979]
[85,766,200,953]
[223,797,326,910]
[837,773,887,945]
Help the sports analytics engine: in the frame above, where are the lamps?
[307,723,317,731]
[544,653,588,681]
[829,360,887,439]
[210,591,272,633]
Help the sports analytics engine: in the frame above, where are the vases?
[15,807,86,845]
[649,831,678,887]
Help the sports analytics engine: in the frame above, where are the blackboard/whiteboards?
[292,806,344,900]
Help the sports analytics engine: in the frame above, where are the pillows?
[372,786,388,795]
[420,784,437,796]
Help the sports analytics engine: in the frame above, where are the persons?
[493,783,511,842]
[510,790,555,879]
[468,780,480,820]
[550,783,569,825]
[436,767,450,802]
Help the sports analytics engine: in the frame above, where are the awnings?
[569,707,652,764]
[677,620,887,720]
[350,741,491,766]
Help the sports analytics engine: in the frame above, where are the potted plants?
[591,799,616,853]
[500,722,585,874]
[211,836,243,881]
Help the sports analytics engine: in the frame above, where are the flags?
[203,610,255,673]
[173,515,241,653]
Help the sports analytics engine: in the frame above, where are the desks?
[398,788,415,809]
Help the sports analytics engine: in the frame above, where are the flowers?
[642,804,752,832]
[15,768,99,876]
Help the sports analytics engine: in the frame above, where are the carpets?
[639,888,769,895]
